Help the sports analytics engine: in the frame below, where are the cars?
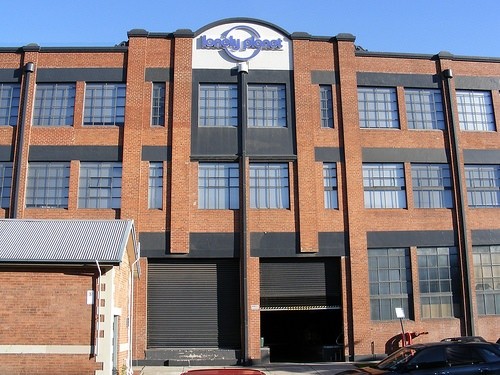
[335,343,500,375]
[440,336,487,342]
